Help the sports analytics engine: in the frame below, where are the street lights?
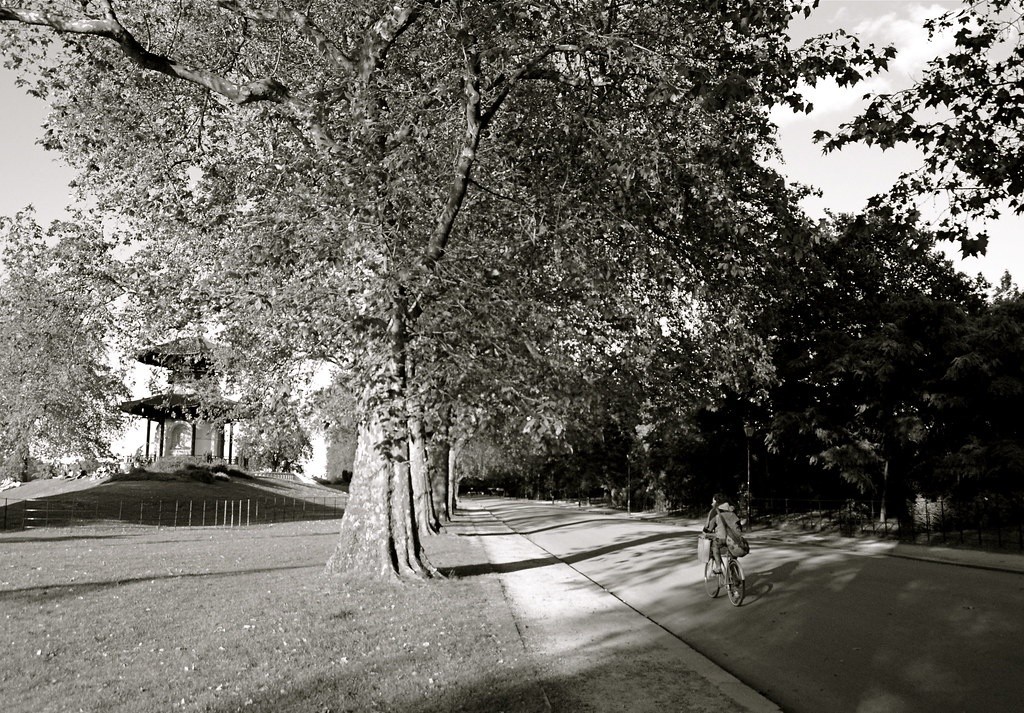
[744,422,755,532]
[626,451,634,516]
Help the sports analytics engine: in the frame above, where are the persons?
[147,458,152,465]
[604,486,617,507]
[41,469,53,480]
[65,469,73,478]
[64,472,67,476]
[154,453,156,461]
[95,463,118,479]
[77,467,87,479]
[202,451,212,464]
[698,493,744,575]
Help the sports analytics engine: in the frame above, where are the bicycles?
[696,535,746,607]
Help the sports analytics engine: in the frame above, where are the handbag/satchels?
[697,537,712,563]
[725,528,749,557]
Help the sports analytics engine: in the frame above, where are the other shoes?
[712,570,722,574]
